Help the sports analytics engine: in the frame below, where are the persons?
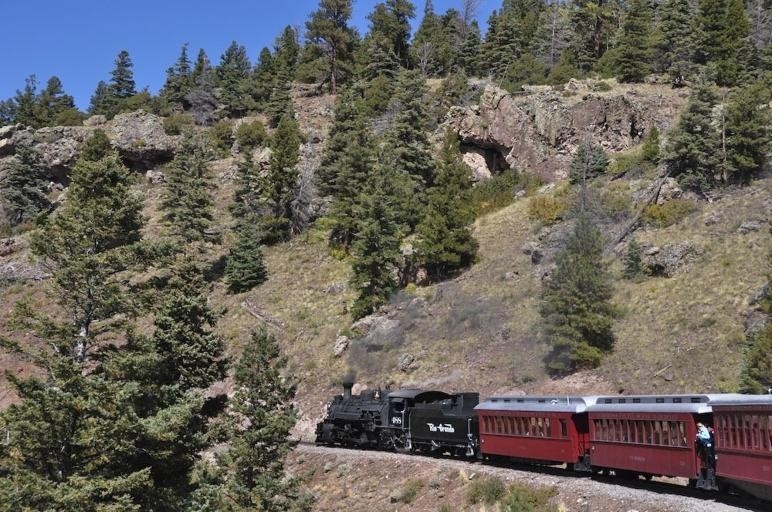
[653,422,716,471]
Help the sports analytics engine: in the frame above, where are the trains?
[314,380,772,500]
